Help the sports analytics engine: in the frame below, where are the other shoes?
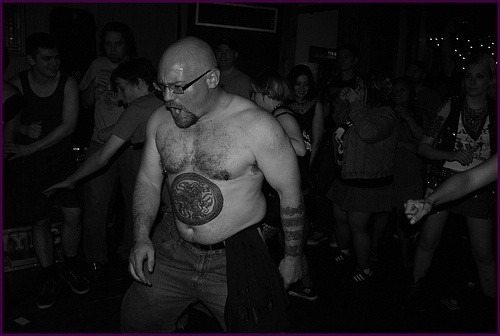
[287,284,318,300]
[59,270,89,294]
[347,270,375,285]
[35,285,58,309]
[84,261,112,285]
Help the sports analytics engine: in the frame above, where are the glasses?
[152,70,211,95]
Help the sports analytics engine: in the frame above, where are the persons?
[0,23,500,308]
[120,36,308,336]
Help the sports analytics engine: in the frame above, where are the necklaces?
[270,104,281,112]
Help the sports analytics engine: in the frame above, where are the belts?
[191,221,263,251]
